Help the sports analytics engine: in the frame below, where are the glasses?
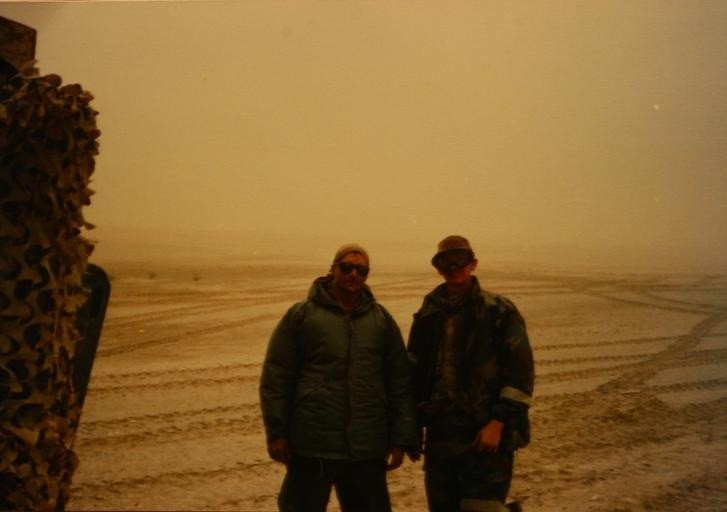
[334,262,369,276]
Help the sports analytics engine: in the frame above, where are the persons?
[407,235,534,511]
[259,242,407,511]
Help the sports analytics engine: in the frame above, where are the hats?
[329,243,369,272]
[432,236,474,268]
